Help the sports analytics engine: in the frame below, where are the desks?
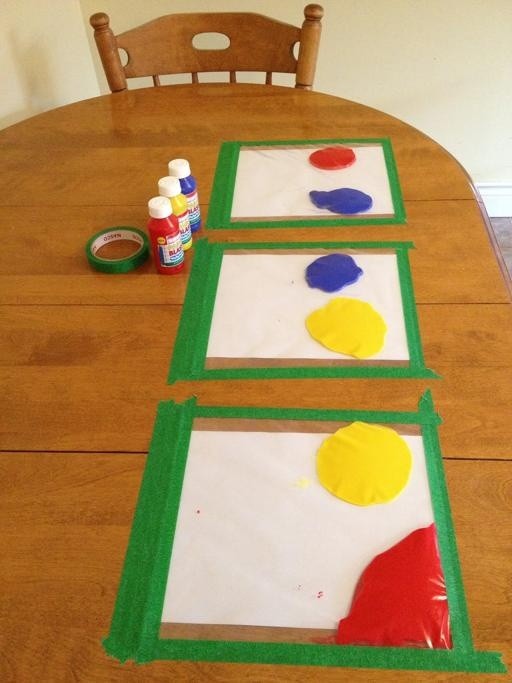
[0,84,511,683]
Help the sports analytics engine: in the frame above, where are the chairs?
[88,1,328,97]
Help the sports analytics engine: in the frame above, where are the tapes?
[86,226,149,273]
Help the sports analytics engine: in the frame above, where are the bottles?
[144,151,201,273]
